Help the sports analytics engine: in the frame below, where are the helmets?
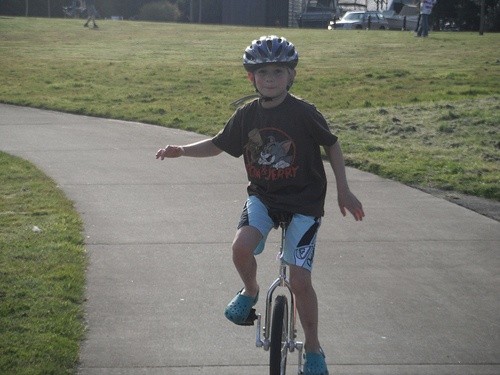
[242,35,299,72]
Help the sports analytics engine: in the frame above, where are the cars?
[327,10,390,31]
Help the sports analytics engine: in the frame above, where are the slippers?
[224,285,259,325]
[302,345,329,375]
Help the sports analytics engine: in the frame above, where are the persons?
[411,0,437,38]
[83,0,99,28]
[154,34,365,375]
